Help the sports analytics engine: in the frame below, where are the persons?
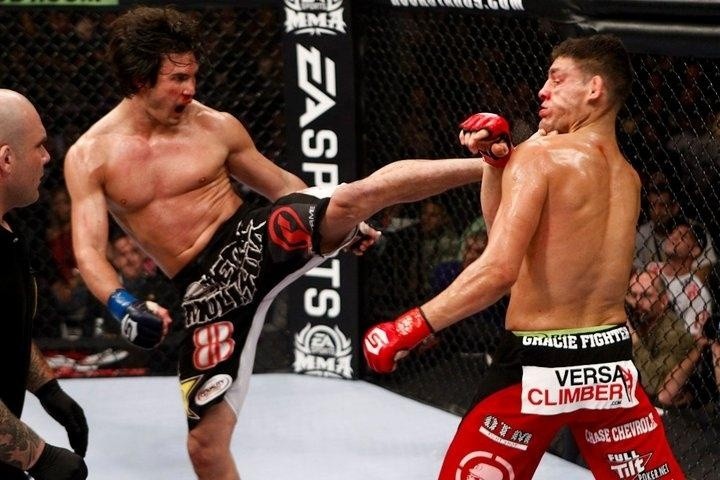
[63,6,559,480]
[0,87,90,480]
[0,187,720,408]
[362,34,685,479]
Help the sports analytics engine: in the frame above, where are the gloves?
[459,113,516,169]
[27,443,88,479]
[106,289,164,350]
[362,306,435,373]
[35,377,87,457]
